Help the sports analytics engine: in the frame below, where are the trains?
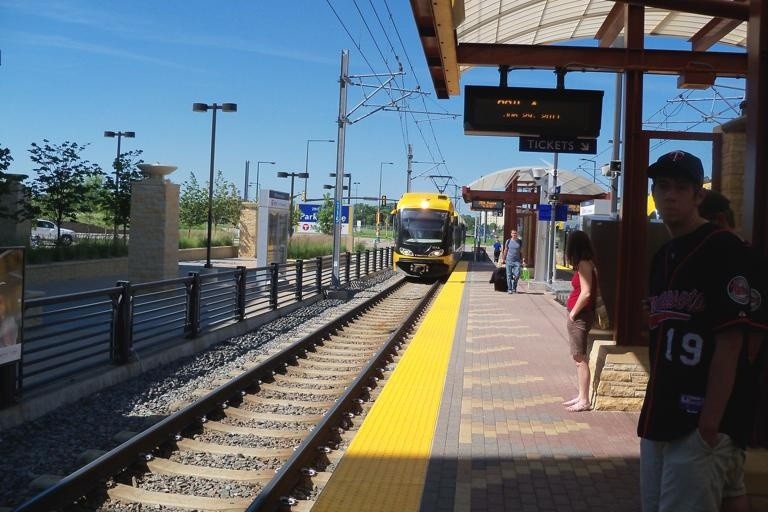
[391,192,467,281]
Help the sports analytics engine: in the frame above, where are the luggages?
[494,263,507,291]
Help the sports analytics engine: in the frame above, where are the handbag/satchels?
[520,264,530,281]
[594,294,610,330]
[490,268,506,283]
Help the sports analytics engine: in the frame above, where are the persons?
[638,150,752,512]
[566,230,596,412]
[700,190,768,511]
[502,228,526,293]
[494,240,501,262]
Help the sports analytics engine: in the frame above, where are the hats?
[643,150,704,178]
[699,189,730,215]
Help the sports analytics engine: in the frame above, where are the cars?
[29,219,76,246]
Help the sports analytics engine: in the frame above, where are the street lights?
[378,161,394,225]
[255,159,276,202]
[323,172,350,236]
[353,182,360,205]
[191,101,239,269]
[303,139,337,203]
[277,171,309,244]
[102,130,136,251]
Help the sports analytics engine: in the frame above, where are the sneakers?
[508,289,515,294]
[561,398,593,411]
